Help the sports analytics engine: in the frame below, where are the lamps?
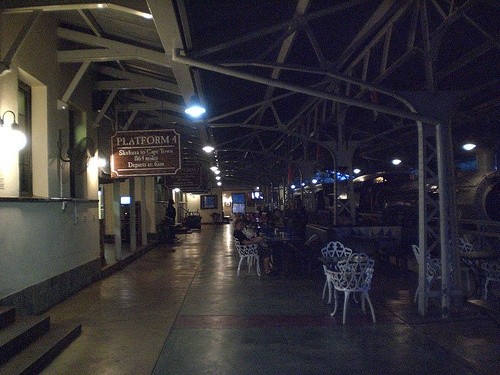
[462,132,476,151]
[0,111,27,149]
[210,162,218,171]
[184,89,205,117]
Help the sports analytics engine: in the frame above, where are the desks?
[240,218,299,276]
[448,252,500,305]
[317,256,379,311]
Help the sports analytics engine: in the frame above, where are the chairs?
[301,233,376,325]
[230,219,261,277]
[410,237,500,303]
[223,211,230,223]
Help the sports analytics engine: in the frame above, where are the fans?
[57,129,95,175]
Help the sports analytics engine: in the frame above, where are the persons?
[234,211,300,275]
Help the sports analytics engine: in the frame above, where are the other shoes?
[265,269,278,276]
